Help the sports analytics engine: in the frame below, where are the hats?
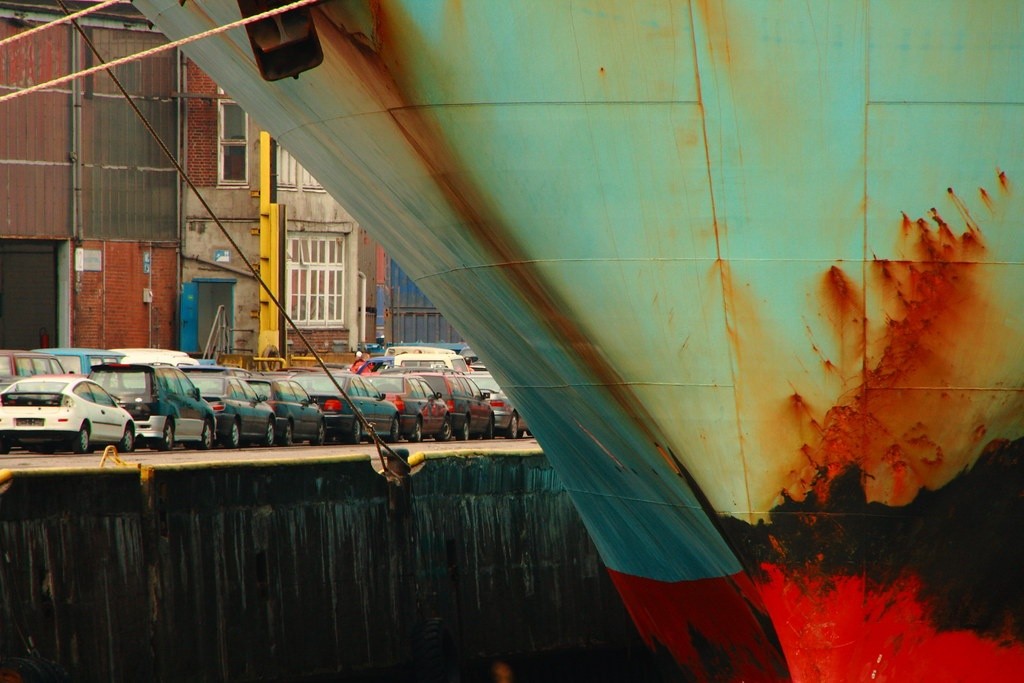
[355,351,362,359]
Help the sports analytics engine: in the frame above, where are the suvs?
[86,362,218,450]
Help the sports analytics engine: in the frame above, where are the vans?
[0,349,127,379]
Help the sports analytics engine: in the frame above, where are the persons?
[464,355,474,373]
[351,353,371,373]
[367,338,385,353]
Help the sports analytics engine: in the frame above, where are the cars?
[0,373,136,456]
[181,345,530,448]
